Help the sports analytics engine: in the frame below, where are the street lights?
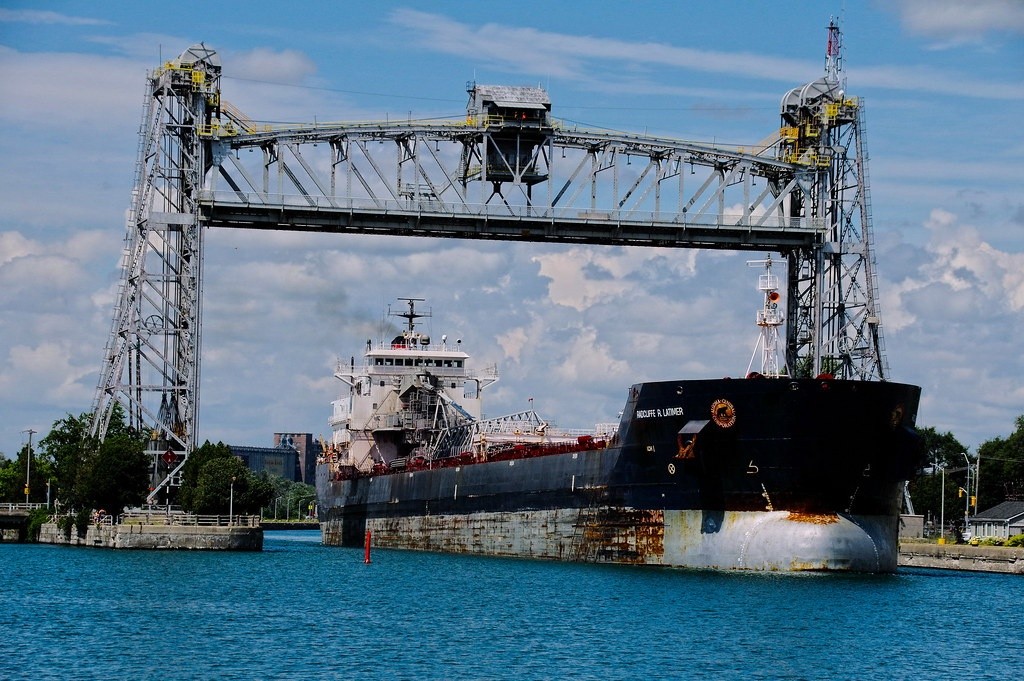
[929,461,948,545]
[958,452,975,533]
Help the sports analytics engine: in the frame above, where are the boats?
[314,299,929,574]
